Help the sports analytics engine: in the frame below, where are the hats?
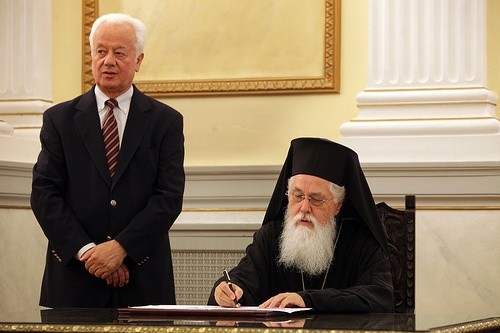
[262,137,390,257]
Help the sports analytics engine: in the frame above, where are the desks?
[0,309,500,333]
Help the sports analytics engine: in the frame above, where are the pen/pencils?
[223,267,239,307]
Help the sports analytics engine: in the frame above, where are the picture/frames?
[81,0,342,100]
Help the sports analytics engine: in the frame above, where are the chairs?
[376,194,416,315]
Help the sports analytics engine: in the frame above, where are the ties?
[101,97,120,183]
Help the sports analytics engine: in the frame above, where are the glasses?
[285,190,347,206]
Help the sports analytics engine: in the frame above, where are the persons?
[207,137,394,312]
[29,13,185,305]
